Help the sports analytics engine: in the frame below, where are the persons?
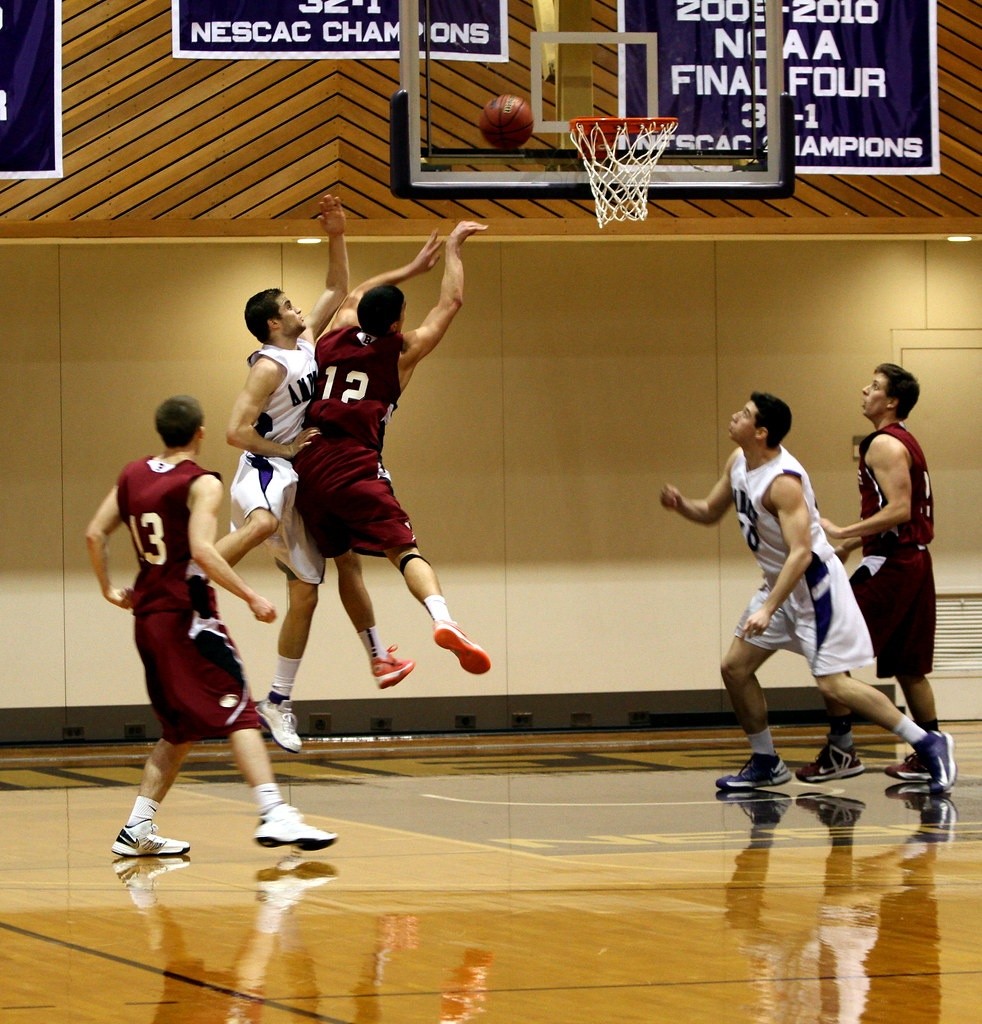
[292,221,491,690]
[114,855,492,1023]
[215,195,349,753]
[87,398,338,854]
[661,392,957,793]
[715,782,957,1024]
[794,363,941,784]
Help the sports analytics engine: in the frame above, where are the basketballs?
[479,94,533,149]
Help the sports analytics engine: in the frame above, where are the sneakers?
[913,730,957,787]
[716,790,793,825]
[796,792,866,830]
[795,743,865,783]
[716,750,792,789]
[254,802,338,851]
[113,856,190,891]
[371,644,415,689]
[432,620,491,675]
[256,860,340,908]
[255,699,302,753]
[885,749,932,781]
[111,819,190,856]
[885,783,958,848]
